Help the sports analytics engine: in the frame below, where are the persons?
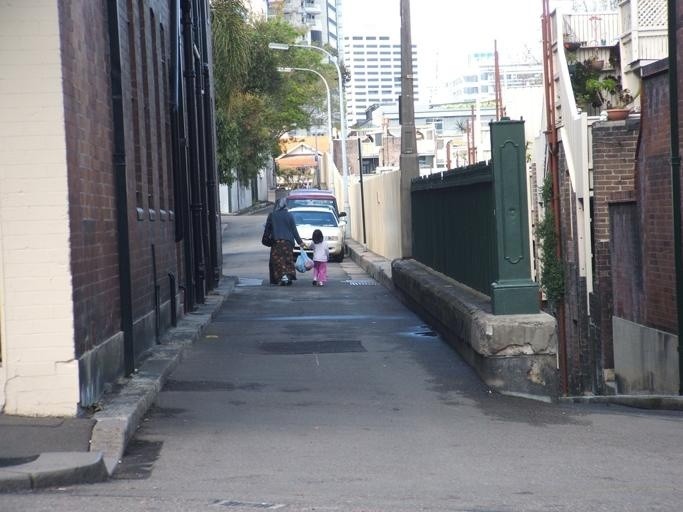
[263,197,305,285]
[304,229,329,287]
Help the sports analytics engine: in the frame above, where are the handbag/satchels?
[261,234,273,247]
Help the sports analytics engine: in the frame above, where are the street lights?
[274,63,336,194]
[267,42,353,239]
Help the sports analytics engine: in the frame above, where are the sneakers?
[312,279,325,286]
[281,280,292,286]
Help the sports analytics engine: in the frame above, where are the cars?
[272,188,348,263]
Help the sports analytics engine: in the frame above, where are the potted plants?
[564,34,581,52]
[589,48,604,72]
[606,101,630,121]
